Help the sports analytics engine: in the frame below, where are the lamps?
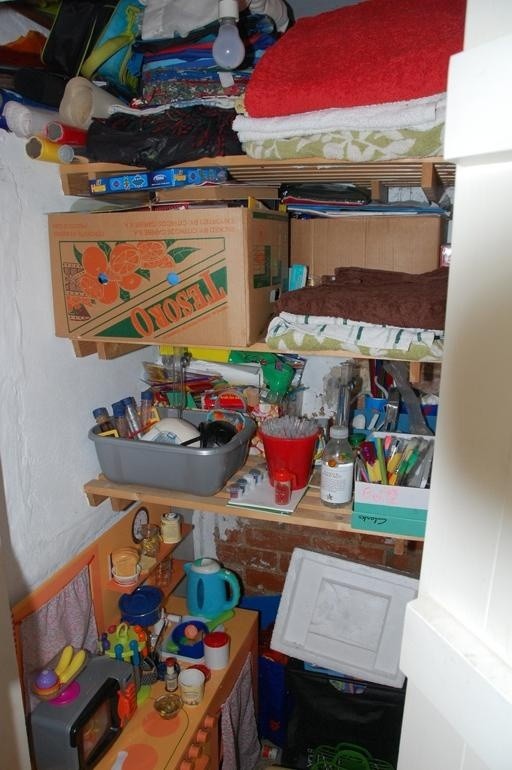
[214,0,246,69]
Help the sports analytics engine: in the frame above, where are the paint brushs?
[381,436,429,486]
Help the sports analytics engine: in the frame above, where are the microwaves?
[22,644,138,770]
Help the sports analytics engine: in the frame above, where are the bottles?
[274,467,292,505]
[203,632,230,670]
[261,744,278,760]
[165,658,178,691]
[91,391,153,439]
[154,559,171,586]
[142,525,159,556]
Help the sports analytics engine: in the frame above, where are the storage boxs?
[47,209,288,349]
[292,218,448,292]
[351,432,435,538]
[89,409,256,495]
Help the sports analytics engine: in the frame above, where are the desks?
[79,595,260,770]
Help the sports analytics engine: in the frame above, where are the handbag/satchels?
[86,104,242,167]
[41,1,146,98]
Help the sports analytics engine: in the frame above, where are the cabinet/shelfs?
[62,155,456,556]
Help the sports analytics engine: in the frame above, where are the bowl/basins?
[154,694,181,718]
[111,564,141,585]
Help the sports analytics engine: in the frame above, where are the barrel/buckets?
[257,424,322,488]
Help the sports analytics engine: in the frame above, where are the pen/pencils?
[353,434,402,485]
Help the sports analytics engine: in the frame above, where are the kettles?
[182,558,240,619]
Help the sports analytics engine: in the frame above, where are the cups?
[112,548,139,575]
[178,669,205,704]
